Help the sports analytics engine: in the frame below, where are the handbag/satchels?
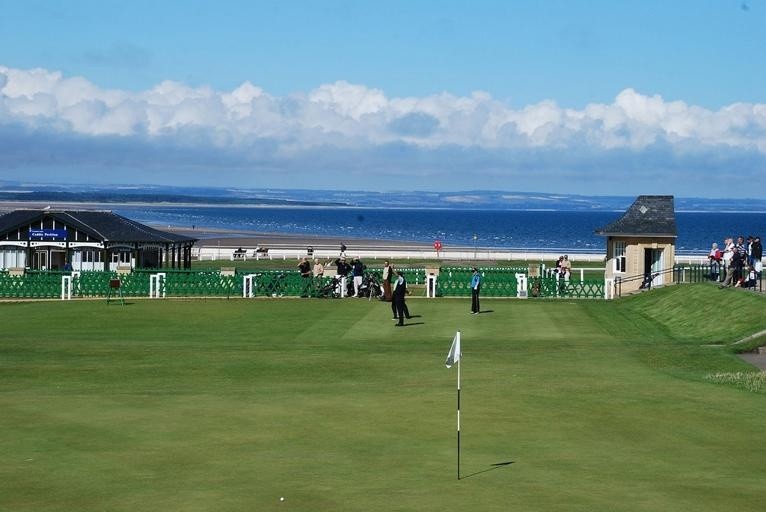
[754,258,763,272]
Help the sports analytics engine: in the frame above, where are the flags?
[445,331,461,368]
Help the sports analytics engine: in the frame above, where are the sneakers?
[340,294,392,301]
[718,284,723,289]
[469,311,480,316]
[391,315,412,326]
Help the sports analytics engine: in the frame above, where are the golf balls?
[280,497,284,502]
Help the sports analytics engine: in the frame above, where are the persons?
[555,256,564,294]
[470,267,481,314]
[382,261,392,298]
[335,256,353,298]
[561,255,571,286]
[340,241,347,258]
[296,257,311,276]
[350,255,367,297]
[324,255,334,265]
[707,234,764,289]
[391,279,411,319]
[311,258,324,278]
[392,271,408,326]
[62,259,74,271]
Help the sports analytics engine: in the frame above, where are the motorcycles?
[311,270,385,300]
